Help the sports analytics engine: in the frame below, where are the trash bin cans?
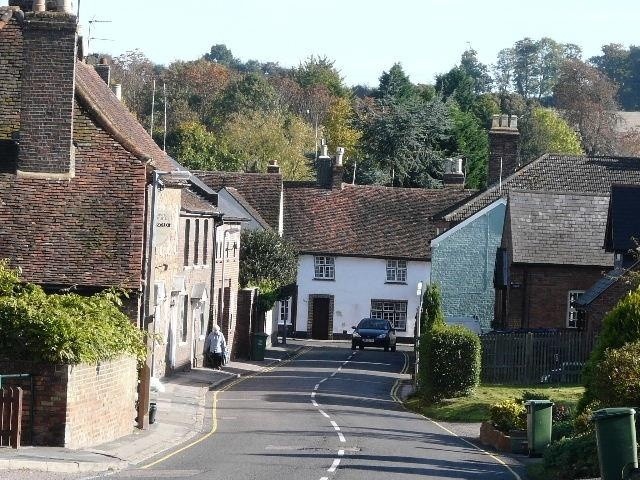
[523,399,555,457]
[591,407,640,480]
[250,332,269,362]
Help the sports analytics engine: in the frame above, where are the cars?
[350,318,398,353]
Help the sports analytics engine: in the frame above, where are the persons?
[206,323,227,370]
